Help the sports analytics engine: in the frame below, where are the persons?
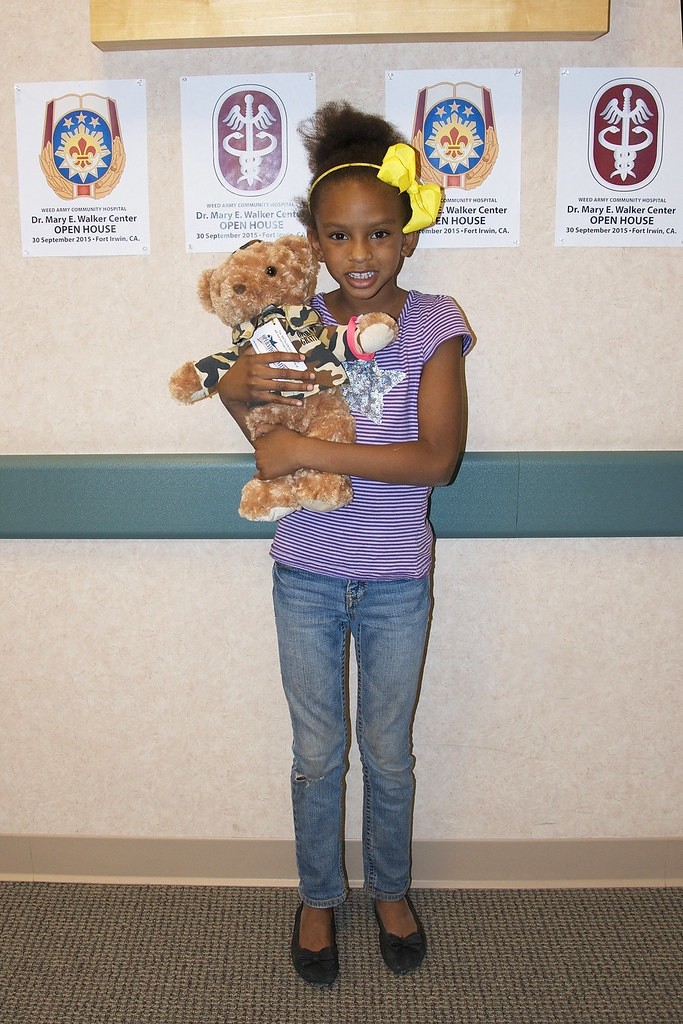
[220,99,475,985]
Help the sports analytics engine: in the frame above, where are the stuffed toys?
[168,235,399,521]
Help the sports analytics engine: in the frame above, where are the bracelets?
[347,315,375,360]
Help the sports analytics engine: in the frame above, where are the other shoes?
[373,892,427,973]
[291,902,339,984]
